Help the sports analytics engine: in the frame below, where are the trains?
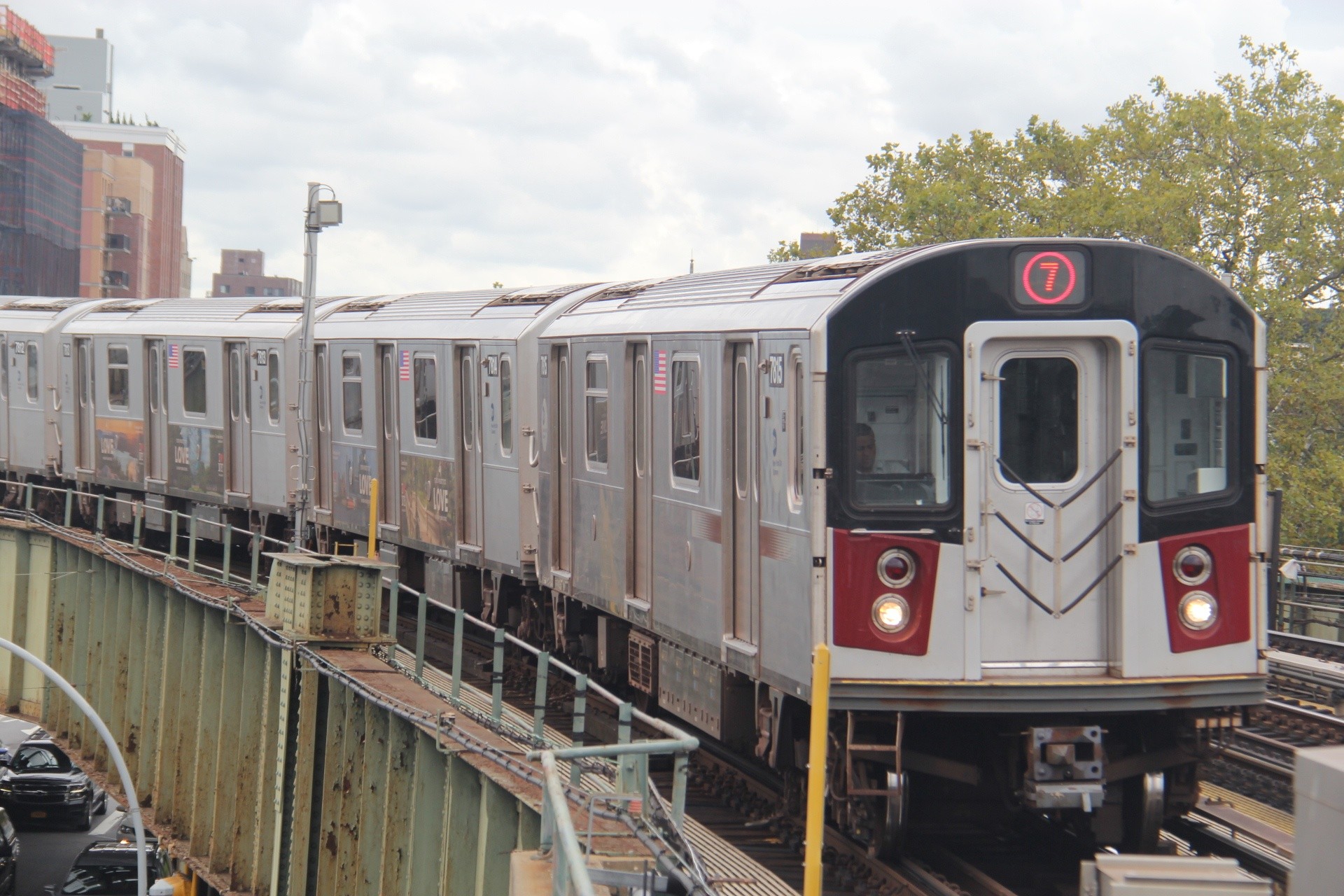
[2,229,1286,889]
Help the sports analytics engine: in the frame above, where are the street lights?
[291,180,344,554]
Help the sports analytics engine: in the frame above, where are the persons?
[852,423,912,497]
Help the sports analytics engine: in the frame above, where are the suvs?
[0,728,111,830]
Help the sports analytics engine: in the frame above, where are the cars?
[59,838,174,896]
[0,807,19,895]
[115,805,159,846]
